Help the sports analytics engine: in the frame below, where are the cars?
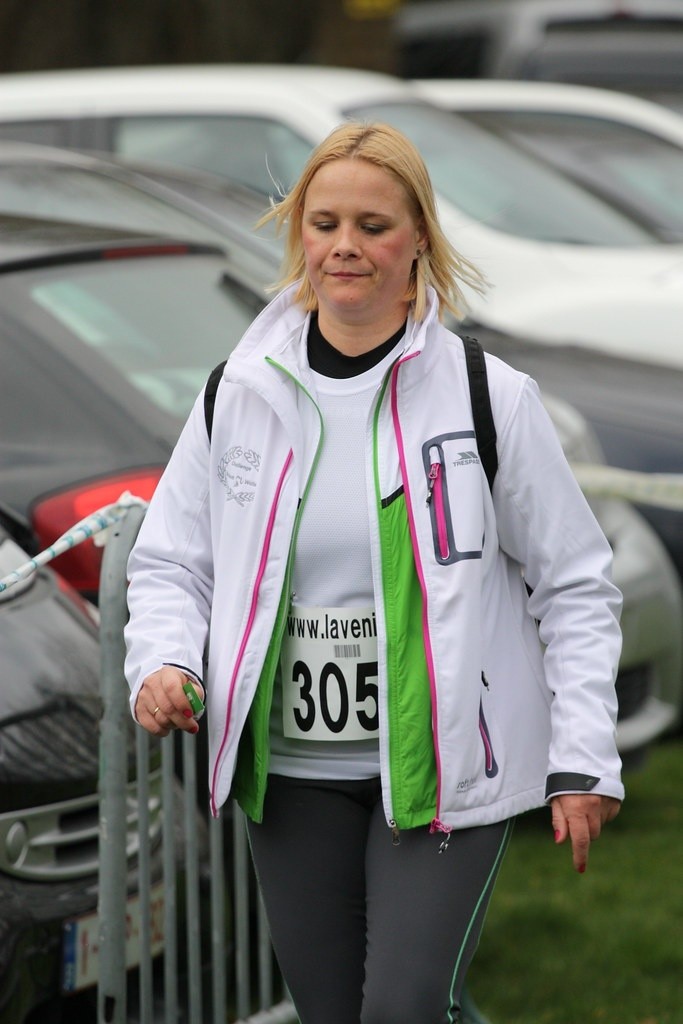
[0,66,682,1024]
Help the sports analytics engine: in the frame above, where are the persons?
[123,122,624,1024]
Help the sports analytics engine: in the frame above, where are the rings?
[153,705,161,716]
